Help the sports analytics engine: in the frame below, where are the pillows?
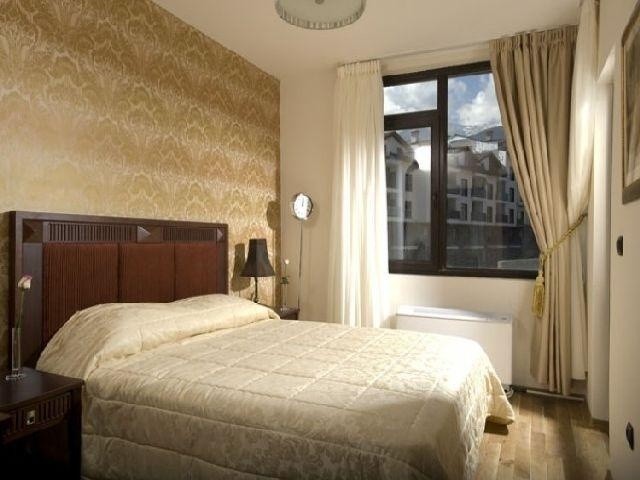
[34,293,281,384]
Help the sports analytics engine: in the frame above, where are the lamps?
[274,0,365,30]
[239,239,276,311]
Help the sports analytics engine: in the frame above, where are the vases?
[280,283,288,311]
[12,327,20,376]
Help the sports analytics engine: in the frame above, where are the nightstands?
[268,306,300,320]
[0,367,84,480]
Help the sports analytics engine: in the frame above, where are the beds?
[9,211,515,480]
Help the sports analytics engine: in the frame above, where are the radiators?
[396,305,512,385]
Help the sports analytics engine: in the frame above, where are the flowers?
[279,258,291,287]
[13,274,32,376]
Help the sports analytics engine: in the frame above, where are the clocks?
[289,192,314,310]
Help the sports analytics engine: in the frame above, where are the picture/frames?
[619,1,640,205]
